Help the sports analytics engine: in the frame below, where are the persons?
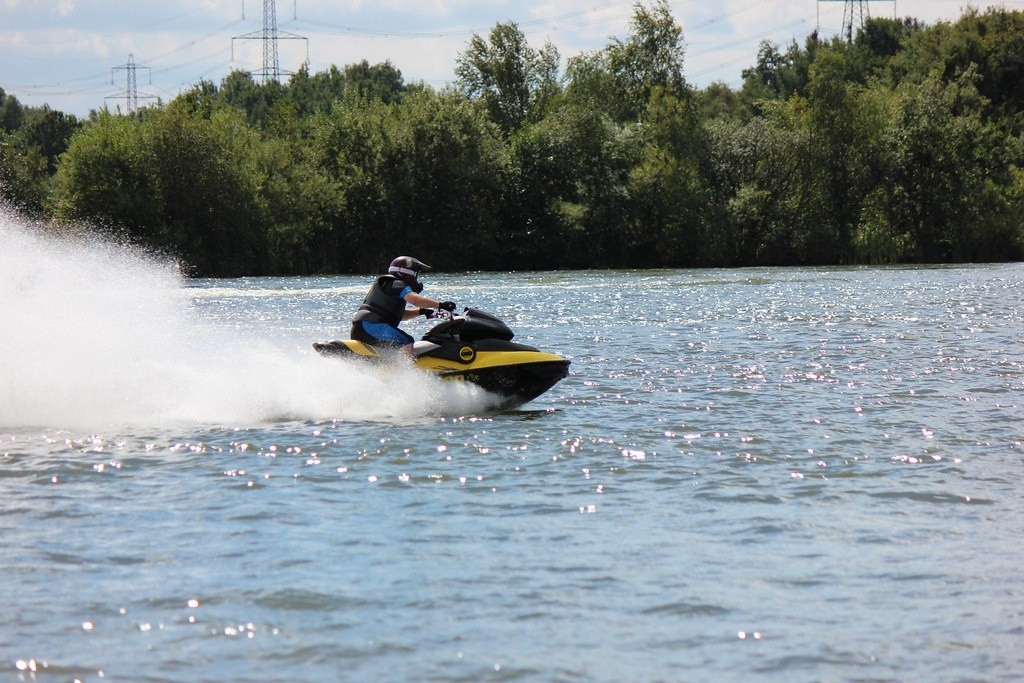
[348,255,458,358]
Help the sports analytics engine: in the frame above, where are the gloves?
[420,308,434,319]
[439,300,456,311]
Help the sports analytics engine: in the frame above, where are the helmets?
[387,256,431,294]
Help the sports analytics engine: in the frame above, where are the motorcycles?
[309,308,571,414]
